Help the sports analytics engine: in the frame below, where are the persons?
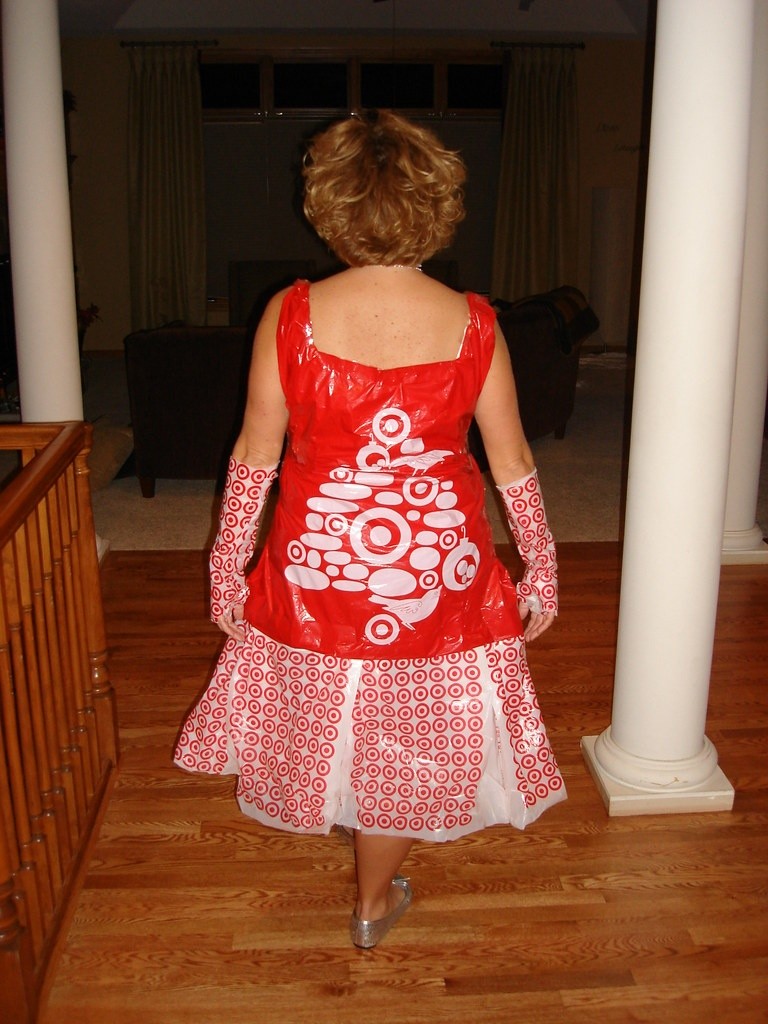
[209,104,560,949]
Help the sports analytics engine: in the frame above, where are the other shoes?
[351,874,411,949]
[337,825,354,842]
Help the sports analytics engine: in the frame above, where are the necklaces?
[379,263,423,272]
